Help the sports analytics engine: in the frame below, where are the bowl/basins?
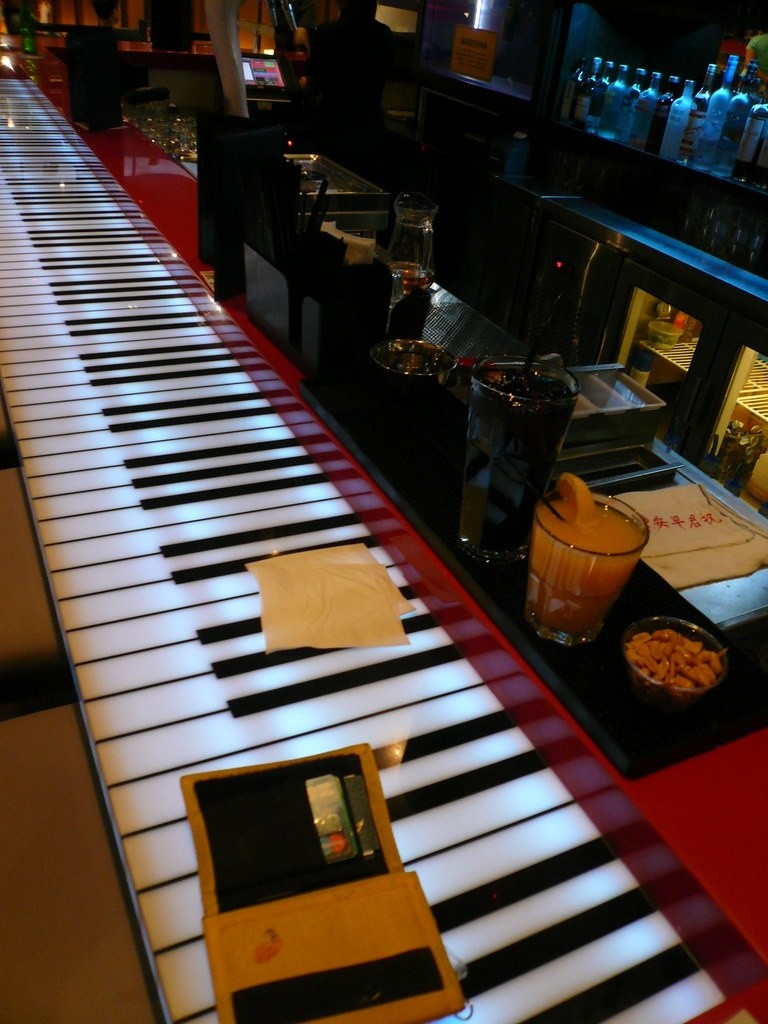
[621,616,730,714]
[370,338,458,401]
[645,320,684,351]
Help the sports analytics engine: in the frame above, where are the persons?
[290,1,405,177]
[91,1,121,27]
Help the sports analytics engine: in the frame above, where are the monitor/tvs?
[240,53,288,98]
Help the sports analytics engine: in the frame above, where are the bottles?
[628,350,653,389]
[17,0,36,55]
[556,54,768,192]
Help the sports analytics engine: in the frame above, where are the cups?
[521,489,651,648]
[382,189,439,294]
[454,354,583,568]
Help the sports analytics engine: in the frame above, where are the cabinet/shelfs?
[591,255,768,517]
[517,220,626,368]
[434,158,536,333]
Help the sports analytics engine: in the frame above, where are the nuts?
[625,628,726,706]
